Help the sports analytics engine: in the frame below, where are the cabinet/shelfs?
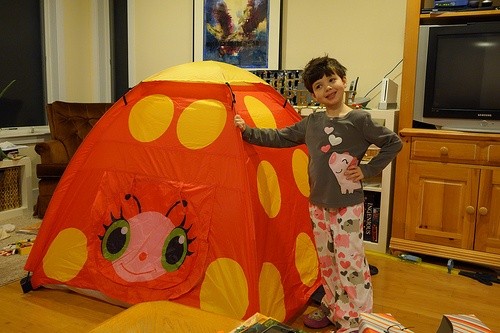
[294,105,395,257]
[389,0,499,270]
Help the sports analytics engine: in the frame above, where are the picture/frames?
[193,1,282,69]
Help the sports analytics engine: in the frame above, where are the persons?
[234,53,403,333]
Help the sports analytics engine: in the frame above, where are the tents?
[18,61,323,328]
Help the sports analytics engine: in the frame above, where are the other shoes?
[304,304,330,328]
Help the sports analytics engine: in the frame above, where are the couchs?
[34,101,113,216]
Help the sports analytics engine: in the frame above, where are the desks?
[87,300,242,333]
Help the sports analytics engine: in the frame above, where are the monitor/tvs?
[413,21,500,134]
